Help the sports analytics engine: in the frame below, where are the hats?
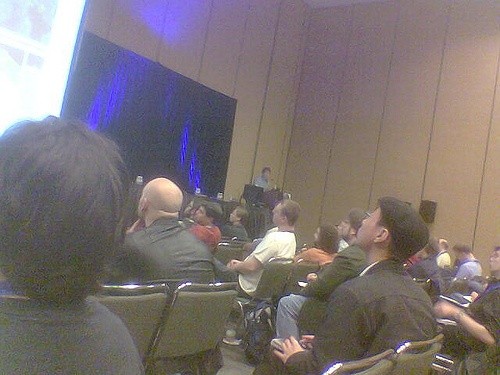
[380,197,430,258]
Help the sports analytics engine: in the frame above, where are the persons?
[443,345,500,375]
[252,196,437,375]
[0,114,145,375]
[433,246,500,371]
[102,167,488,346]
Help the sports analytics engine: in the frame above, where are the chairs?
[94,237,491,375]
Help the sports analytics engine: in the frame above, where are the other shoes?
[223,337,242,345]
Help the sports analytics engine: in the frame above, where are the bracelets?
[453,308,466,324]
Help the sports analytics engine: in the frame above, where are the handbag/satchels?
[245,314,275,365]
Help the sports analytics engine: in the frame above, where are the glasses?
[274,202,282,216]
[361,212,381,226]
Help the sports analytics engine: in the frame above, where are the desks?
[130,184,273,242]
